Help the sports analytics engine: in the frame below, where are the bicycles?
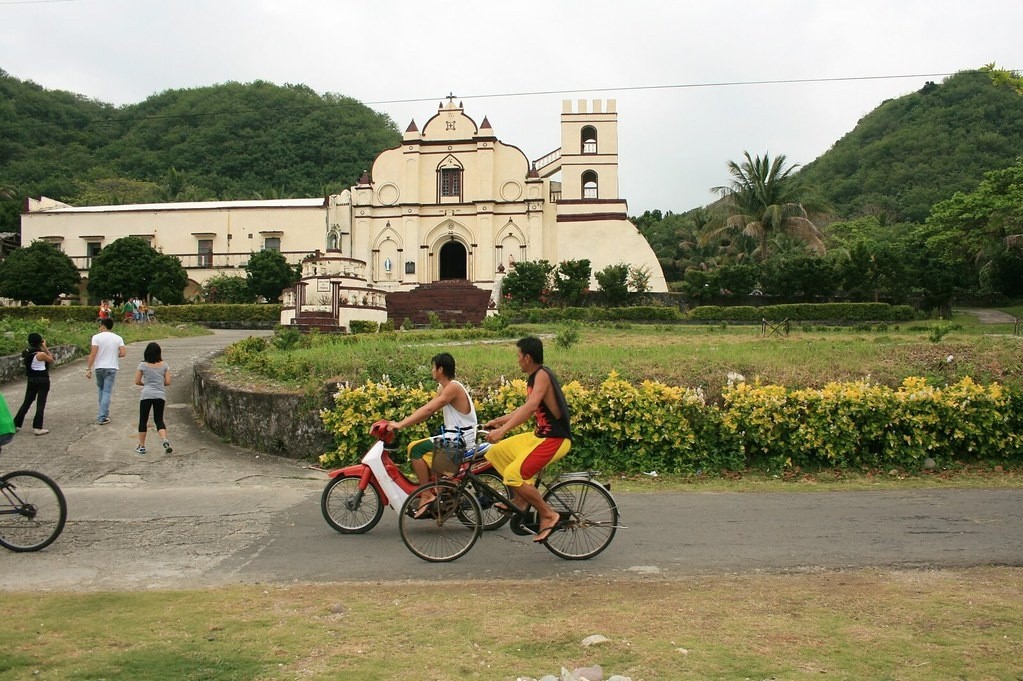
[0,433,67,552]
[122,305,156,324]
[399,425,628,562]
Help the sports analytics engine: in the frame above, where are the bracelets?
[87,368,91,371]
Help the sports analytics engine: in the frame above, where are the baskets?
[147,308,154,315]
[431,438,467,477]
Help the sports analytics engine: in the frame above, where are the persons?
[135,342,173,453]
[483,337,571,543]
[86,318,126,424]
[13,333,53,435]
[387,354,477,518]
[121,296,142,324]
[0,394,16,448]
[99,301,112,319]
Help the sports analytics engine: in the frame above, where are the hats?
[28,333,42,346]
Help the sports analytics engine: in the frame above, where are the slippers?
[534,518,562,543]
[494,505,520,514]
[414,498,437,519]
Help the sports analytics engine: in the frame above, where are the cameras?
[41,340,44,346]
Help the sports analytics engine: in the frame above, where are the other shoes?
[15,427,19,432]
[135,445,146,454]
[100,418,110,425]
[34,428,49,436]
[162,439,172,452]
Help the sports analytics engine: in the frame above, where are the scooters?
[321,419,514,534]
